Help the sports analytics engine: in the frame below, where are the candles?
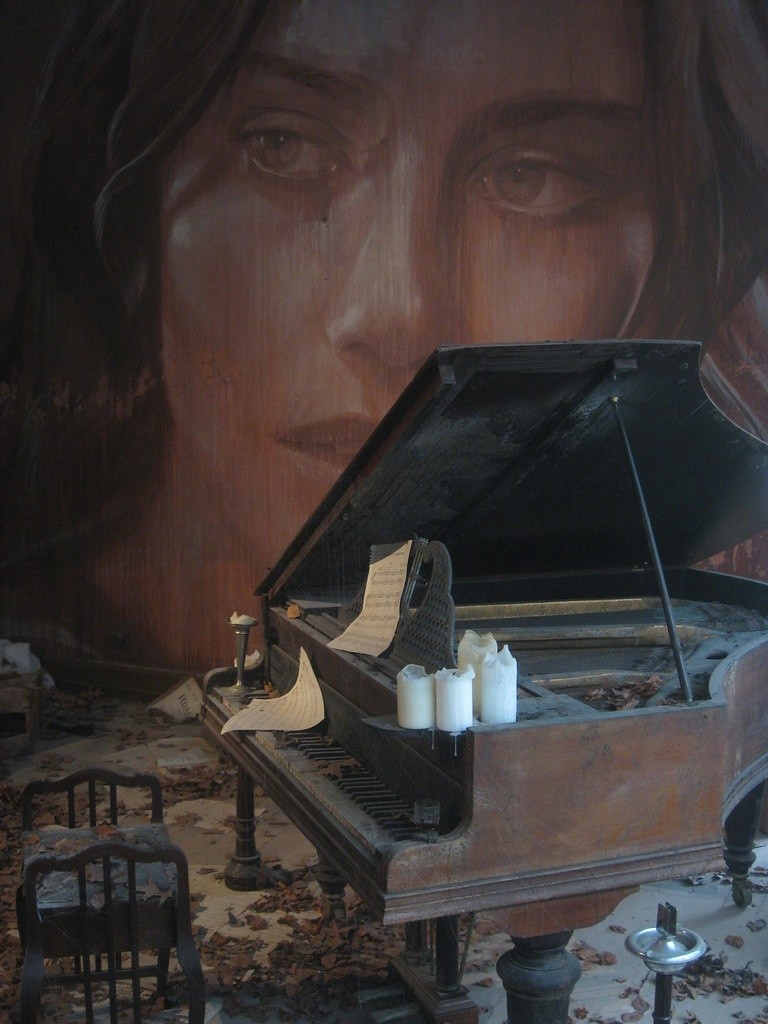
[480,645,517,724]
[457,629,498,713]
[230,611,254,624]
[397,665,436,729]
[436,664,472,731]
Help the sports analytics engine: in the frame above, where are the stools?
[16,769,205,1024]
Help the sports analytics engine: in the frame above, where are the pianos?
[196,337,768,1024]
